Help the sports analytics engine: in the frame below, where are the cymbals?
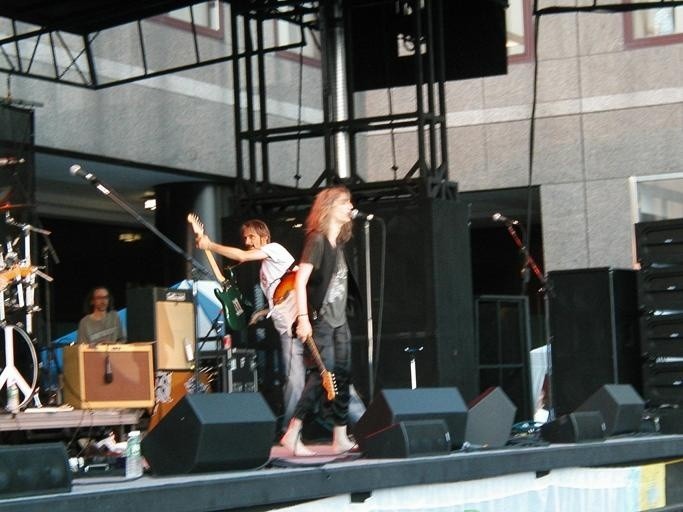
[0,202,33,208]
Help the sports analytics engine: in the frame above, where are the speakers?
[540,410,608,442]
[140,390,279,476]
[571,382,648,437]
[0,441,73,501]
[125,285,197,371]
[350,386,469,457]
[465,385,519,449]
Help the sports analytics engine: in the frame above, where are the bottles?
[126,433,140,477]
[131,430,142,476]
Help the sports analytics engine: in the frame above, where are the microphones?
[103,355,115,384]
[346,208,375,225]
[493,211,520,229]
[68,161,100,188]
[5,216,26,230]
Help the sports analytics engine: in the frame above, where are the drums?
[1,324,38,416]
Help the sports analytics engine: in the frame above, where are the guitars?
[272,270,338,400]
[187,212,254,331]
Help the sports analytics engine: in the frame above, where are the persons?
[76,284,126,347]
[281,185,361,458]
[196,218,310,440]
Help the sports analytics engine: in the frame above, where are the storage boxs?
[63,344,154,409]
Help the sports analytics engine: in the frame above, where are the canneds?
[223,335,233,351]
